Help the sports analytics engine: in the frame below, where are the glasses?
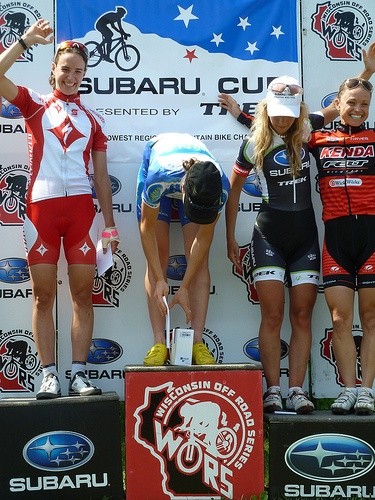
[269,83,302,96]
[55,41,89,60]
[337,79,374,97]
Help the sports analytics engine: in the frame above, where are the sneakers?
[67,371,101,395]
[330,388,357,413]
[262,390,283,413]
[353,388,375,414]
[192,342,215,364]
[285,389,314,412]
[142,343,167,366]
[36,372,62,398]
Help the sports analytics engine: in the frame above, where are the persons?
[218,78,375,415]
[226,41,375,412]
[135,132,230,366]
[0,18,121,399]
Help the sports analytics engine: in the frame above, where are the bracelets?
[17,36,29,51]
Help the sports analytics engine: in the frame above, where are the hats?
[266,75,301,118]
[183,163,222,223]
